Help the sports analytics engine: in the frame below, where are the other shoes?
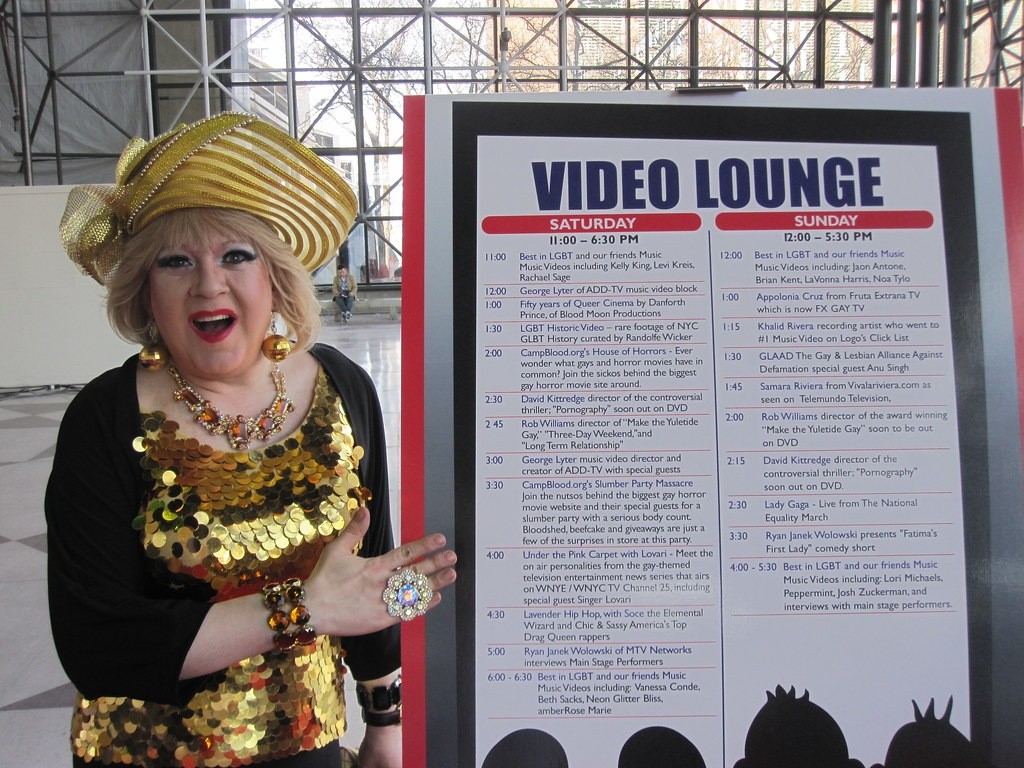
[340,311,352,320]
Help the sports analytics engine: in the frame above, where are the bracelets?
[263,577,317,651]
[356,675,402,710]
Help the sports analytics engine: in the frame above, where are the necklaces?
[167,363,296,449]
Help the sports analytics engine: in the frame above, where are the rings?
[382,570,432,622]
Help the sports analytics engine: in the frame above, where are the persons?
[45,111,461,768]
[333,265,356,322]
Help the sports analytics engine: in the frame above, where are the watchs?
[361,705,402,726]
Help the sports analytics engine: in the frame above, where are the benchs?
[318,298,402,320]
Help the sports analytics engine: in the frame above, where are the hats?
[59,110,359,288]
